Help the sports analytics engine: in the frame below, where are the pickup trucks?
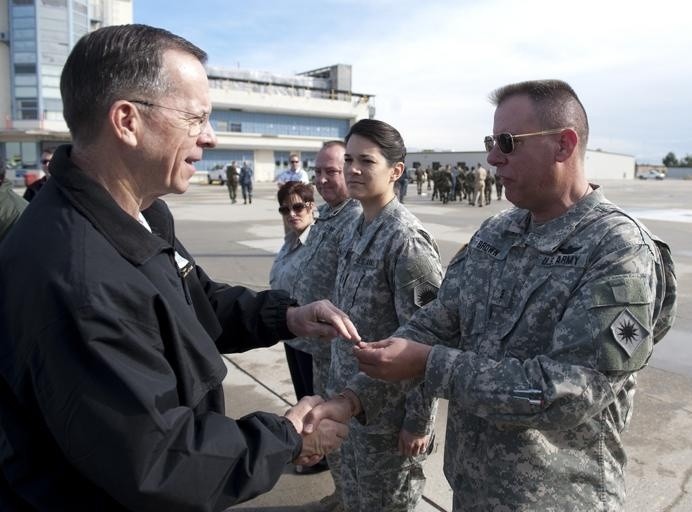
[635,170,666,181]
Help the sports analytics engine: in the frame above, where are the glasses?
[127,99,210,127]
[278,202,311,215]
[483,127,566,158]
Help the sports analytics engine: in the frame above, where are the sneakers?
[292,463,329,477]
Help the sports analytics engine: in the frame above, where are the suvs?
[208,162,243,186]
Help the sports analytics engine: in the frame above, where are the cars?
[407,167,429,184]
[306,167,315,181]
[16,163,39,179]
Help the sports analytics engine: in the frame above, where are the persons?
[292,79,678,510]
[298,140,364,512]
[265,177,330,476]
[0,24,361,511]
[20,148,57,205]
[225,159,241,205]
[0,152,31,245]
[321,118,445,511]
[276,152,310,234]
[393,162,504,208]
[238,158,254,206]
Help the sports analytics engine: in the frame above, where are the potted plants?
[4,155,22,187]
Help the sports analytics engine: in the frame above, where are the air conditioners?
[0,32,9,42]
